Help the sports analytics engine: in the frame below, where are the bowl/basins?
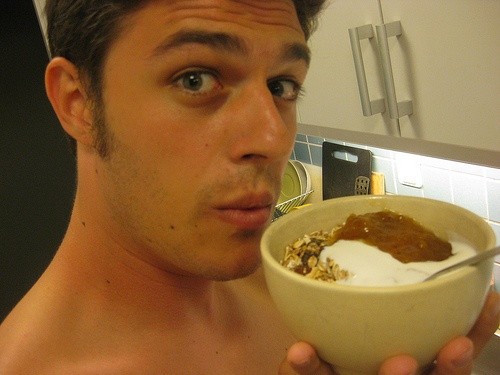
[259,193,495,375]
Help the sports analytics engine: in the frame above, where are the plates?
[280,158,310,207]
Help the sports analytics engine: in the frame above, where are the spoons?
[392,245,500,285]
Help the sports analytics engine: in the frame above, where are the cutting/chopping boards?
[321,140,372,201]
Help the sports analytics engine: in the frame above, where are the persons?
[0,0,500,375]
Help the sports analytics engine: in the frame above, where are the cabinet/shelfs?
[33,0,500,169]
